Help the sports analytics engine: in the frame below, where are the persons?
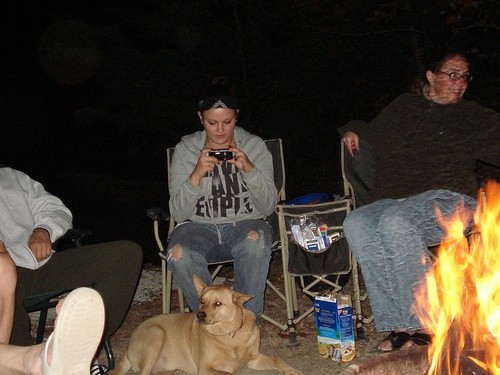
[0,254,105,375]
[0,168,143,375]
[165,93,280,325]
[341,49,500,352]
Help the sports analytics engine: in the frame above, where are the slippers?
[40,286,106,375]
[399,331,432,352]
[375,331,411,352]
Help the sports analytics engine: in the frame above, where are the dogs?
[106,273,304,375]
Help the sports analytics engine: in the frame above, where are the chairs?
[341,138,481,340]
[25,228,115,372]
[147,138,299,338]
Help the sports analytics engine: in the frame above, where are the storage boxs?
[313,292,356,364]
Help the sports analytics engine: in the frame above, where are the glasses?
[439,70,473,83]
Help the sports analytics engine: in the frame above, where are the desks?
[274,194,365,343]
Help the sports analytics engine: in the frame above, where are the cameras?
[209,148,233,161]
